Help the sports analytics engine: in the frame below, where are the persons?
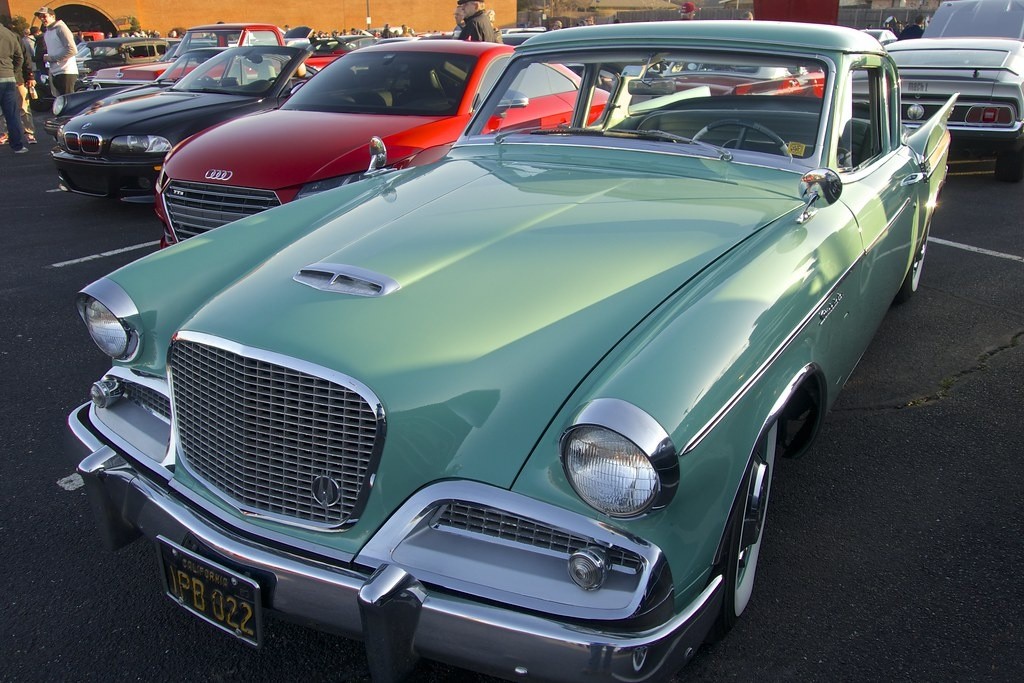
[74,31,86,45]
[740,12,753,21]
[449,0,504,44]
[311,23,414,38]
[105,29,179,38]
[613,19,619,23]
[284,24,290,32]
[0,8,79,152]
[898,15,925,41]
[884,21,909,36]
[678,2,699,19]
[553,20,563,30]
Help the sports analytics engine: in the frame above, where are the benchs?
[637,108,874,168]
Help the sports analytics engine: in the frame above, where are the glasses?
[38,15,46,19]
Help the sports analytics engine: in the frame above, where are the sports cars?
[155,39,610,248]
[47,45,322,195]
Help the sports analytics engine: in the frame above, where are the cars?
[65,18,965,683]
[70,0,1023,163]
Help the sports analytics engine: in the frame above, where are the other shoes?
[0,132,9,145]
[15,147,29,154]
[24,132,37,144]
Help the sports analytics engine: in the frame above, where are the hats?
[34,7,56,16]
[28,26,39,36]
[458,0,484,5]
[681,2,701,13]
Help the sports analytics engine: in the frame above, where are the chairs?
[281,61,308,91]
[394,67,445,109]
